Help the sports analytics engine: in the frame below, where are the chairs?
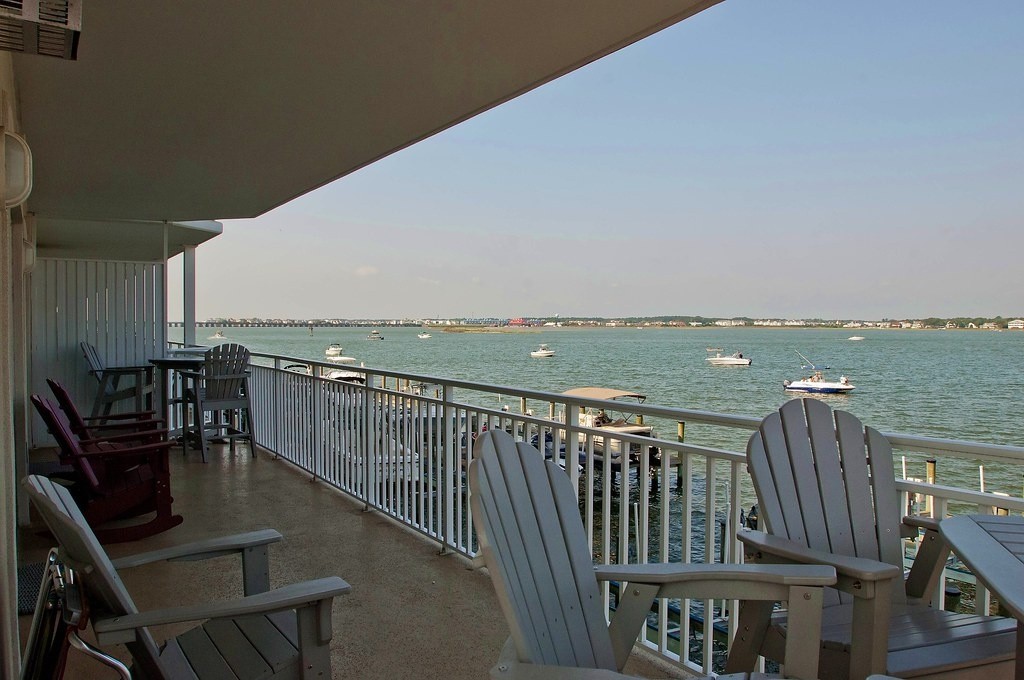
[467,429,836,680]
[177,344,257,463]
[30,394,184,544]
[47,379,165,482]
[19,474,352,680]
[80,342,156,428]
[737,398,1020,680]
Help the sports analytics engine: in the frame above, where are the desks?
[148,358,228,450]
[938,512,1024,680]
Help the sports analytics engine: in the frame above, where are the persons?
[800,373,825,383]
[717,350,743,359]
[840,375,849,387]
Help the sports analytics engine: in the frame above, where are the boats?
[326,344,342,355]
[308,392,468,485]
[418,331,432,338]
[785,381,856,393]
[214,331,227,340]
[705,357,752,365]
[562,388,654,442]
[366,329,384,340]
[323,357,365,384]
[531,343,555,357]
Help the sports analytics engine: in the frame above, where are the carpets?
[19,562,46,615]
